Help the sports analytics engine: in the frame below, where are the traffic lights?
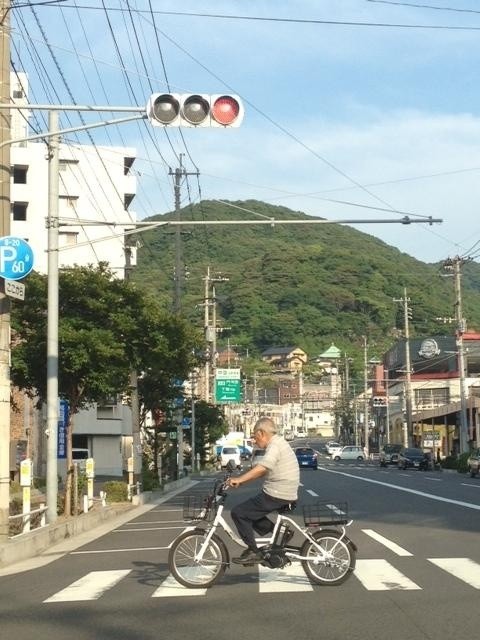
[372,396,387,407]
[147,93,244,128]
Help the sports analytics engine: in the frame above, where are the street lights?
[446,257,467,456]
[189,325,215,472]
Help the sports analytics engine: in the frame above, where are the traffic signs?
[0,236,34,280]
[215,369,240,401]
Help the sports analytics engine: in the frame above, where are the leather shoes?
[274,529,294,546]
[232,548,265,564]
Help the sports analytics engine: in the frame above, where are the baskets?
[304,502,349,523]
[182,494,209,520]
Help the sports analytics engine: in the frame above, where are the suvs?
[379,444,405,467]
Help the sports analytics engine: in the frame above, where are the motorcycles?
[167,473,358,588]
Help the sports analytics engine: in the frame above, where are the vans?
[215,441,253,470]
[333,446,364,461]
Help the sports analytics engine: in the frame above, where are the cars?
[399,448,428,470]
[296,430,305,438]
[326,441,341,455]
[466,447,480,477]
[294,447,317,470]
[286,431,294,441]
[251,448,266,467]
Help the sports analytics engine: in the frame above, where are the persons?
[437,448,443,470]
[217,452,222,470]
[229,418,300,565]
[363,443,370,467]
